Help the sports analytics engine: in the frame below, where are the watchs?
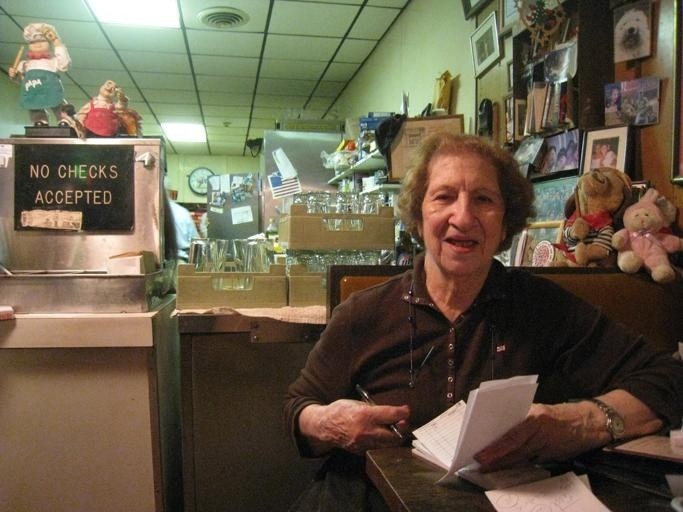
[581,394,627,445]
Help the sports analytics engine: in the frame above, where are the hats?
[374,114,407,171]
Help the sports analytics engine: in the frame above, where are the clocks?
[189,166,217,196]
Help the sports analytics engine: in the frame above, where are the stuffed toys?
[548,166,632,268]
[610,187,682,284]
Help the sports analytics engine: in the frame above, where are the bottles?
[266,218,278,241]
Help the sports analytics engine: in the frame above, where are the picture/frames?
[516,220,566,268]
[529,126,581,178]
[462,0,526,147]
[578,125,630,181]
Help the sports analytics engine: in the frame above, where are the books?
[410,399,551,493]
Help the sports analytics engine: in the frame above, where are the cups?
[289,246,382,286]
[189,237,268,290]
[293,191,387,231]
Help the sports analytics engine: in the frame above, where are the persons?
[605,86,654,125]
[532,137,578,177]
[600,143,617,170]
[590,143,603,169]
[8,23,73,127]
[278,130,682,511]
[75,80,129,137]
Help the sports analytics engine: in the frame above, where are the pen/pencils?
[355,383,403,439]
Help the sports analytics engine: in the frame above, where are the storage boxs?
[175,203,396,309]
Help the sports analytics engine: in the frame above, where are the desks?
[363,434,683,512]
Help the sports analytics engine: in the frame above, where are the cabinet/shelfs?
[513,0,611,146]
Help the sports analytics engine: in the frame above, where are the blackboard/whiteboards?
[14,143,135,232]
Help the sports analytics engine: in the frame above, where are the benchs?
[325,262,683,512]
